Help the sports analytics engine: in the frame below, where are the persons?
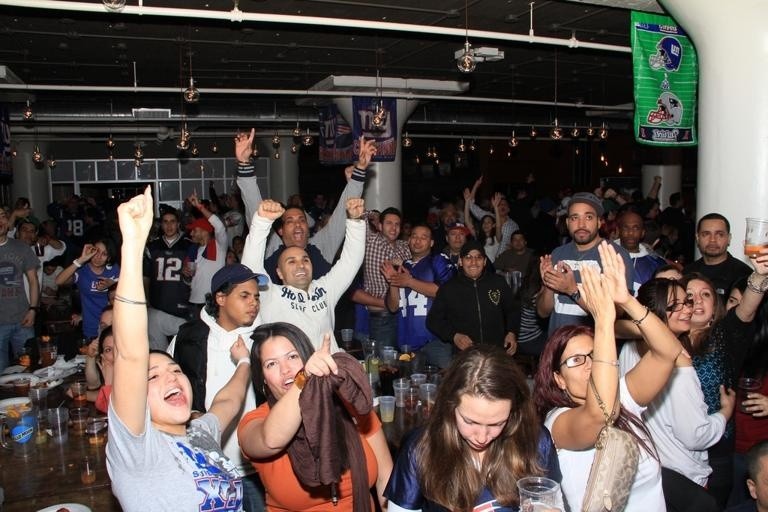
[0,127,768,512]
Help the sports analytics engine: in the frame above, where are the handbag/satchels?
[581,425,640,511]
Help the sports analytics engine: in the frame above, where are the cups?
[35,240,45,257]
[737,378,762,413]
[340,328,354,342]
[37,336,57,368]
[0,409,36,456]
[361,338,397,386]
[79,455,97,484]
[17,346,32,367]
[79,337,88,355]
[379,365,442,423]
[187,261,197,276]
[400,344,412,361]
[29,382,105,445]
[515,475,564,511]
[743,216,768,258]
[13,376,31,397]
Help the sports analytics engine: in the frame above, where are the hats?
[567,192,604,216]
[447,223,485,256]
[184,217,214,232]
[212,263,269,294]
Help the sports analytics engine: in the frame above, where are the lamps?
[181,42,202,103]
[455,1,481,74]
[101,0,127,11]
[4,100,627,181]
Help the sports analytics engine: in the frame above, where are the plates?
[35,365,78,379]
[0,373,38,387]
[0,395,32,413]
[36,503,91,512]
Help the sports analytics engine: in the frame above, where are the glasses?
[667,299,693,312]
[559,351,593,368]
